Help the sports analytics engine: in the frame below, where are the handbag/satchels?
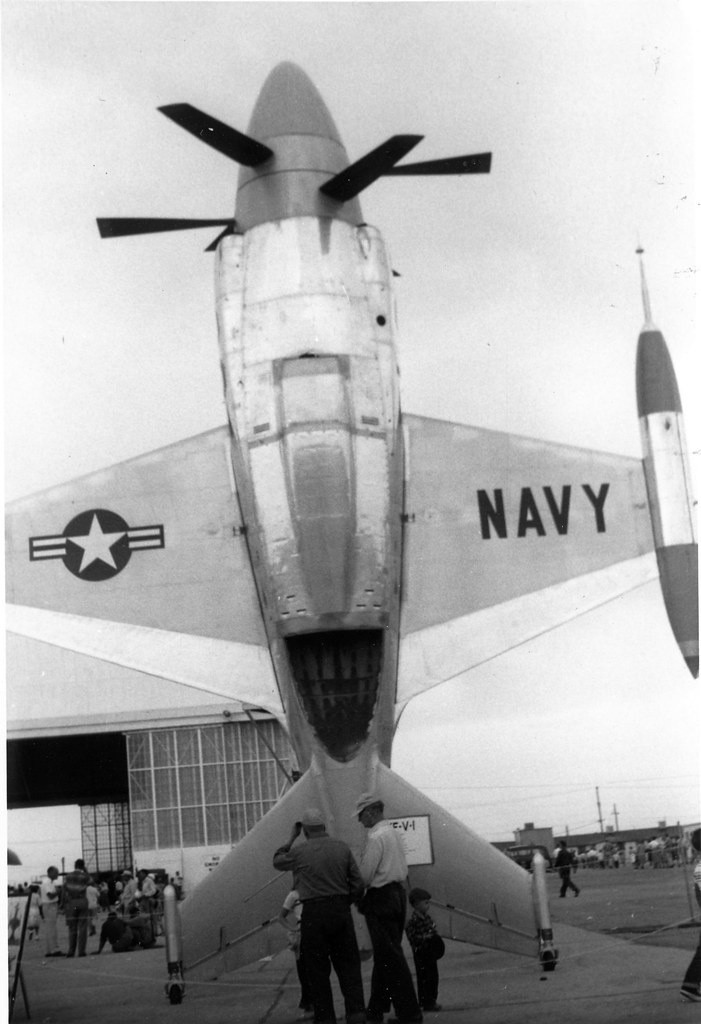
[286,931,300,951]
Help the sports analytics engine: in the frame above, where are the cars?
[503,844,553,870]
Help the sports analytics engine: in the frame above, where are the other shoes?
[419,1001,441,1012]
[29,932,33,941]
[365,1008,383,1022]
[575,889,579,897]
[388,1017,422,1024]
[45,951,67,957]
[303,1009,314,1020]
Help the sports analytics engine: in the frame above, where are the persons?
[552,828,700,874]
[272,808,364,1024]
[41,867,61,957]
[554,841,581,899]
[679,828,701,1002]
[350,792,426,1024]
[278,881,313,1017]
[91,910,156,955]
[406,887,445,1010]
[11,834,186,923]
[62,858,92,956]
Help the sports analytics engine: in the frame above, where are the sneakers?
[680,985,701,1000]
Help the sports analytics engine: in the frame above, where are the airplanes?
[4,61,698,1007]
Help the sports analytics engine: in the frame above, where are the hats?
[121,871,131,875]
[301,808,325,825]
[353,793,380,817]
[430,934,445,961]
